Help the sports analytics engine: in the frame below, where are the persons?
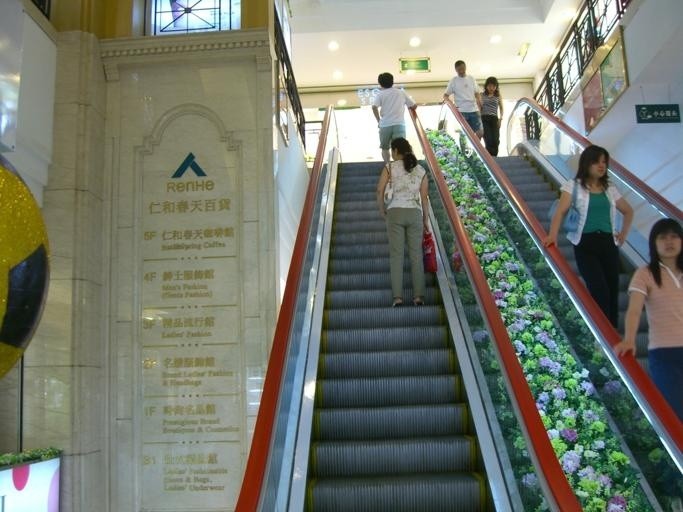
[540,143,633,330]
[444,60,485,154]
[371,70,417,163]
[473,76,504,158]
[377,135,438,305]
[613,216,682,421]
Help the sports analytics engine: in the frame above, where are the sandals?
[393,299,424,306]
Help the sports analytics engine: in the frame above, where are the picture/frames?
[579,25,629,136]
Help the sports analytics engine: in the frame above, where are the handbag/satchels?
[423,231,437,273]
[384,163,393,204]
[547,179,580,232]
